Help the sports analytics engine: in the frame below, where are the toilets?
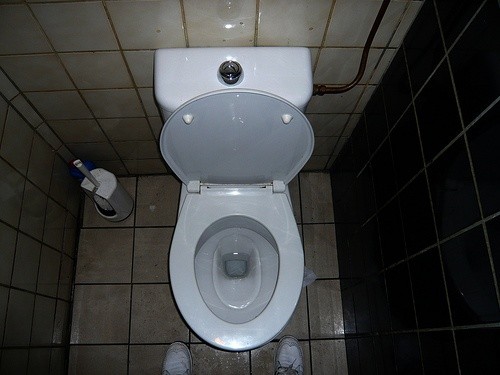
[154,46,314,353]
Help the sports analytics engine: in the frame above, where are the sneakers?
[273,335,304,375]
[161,341,192,375]
